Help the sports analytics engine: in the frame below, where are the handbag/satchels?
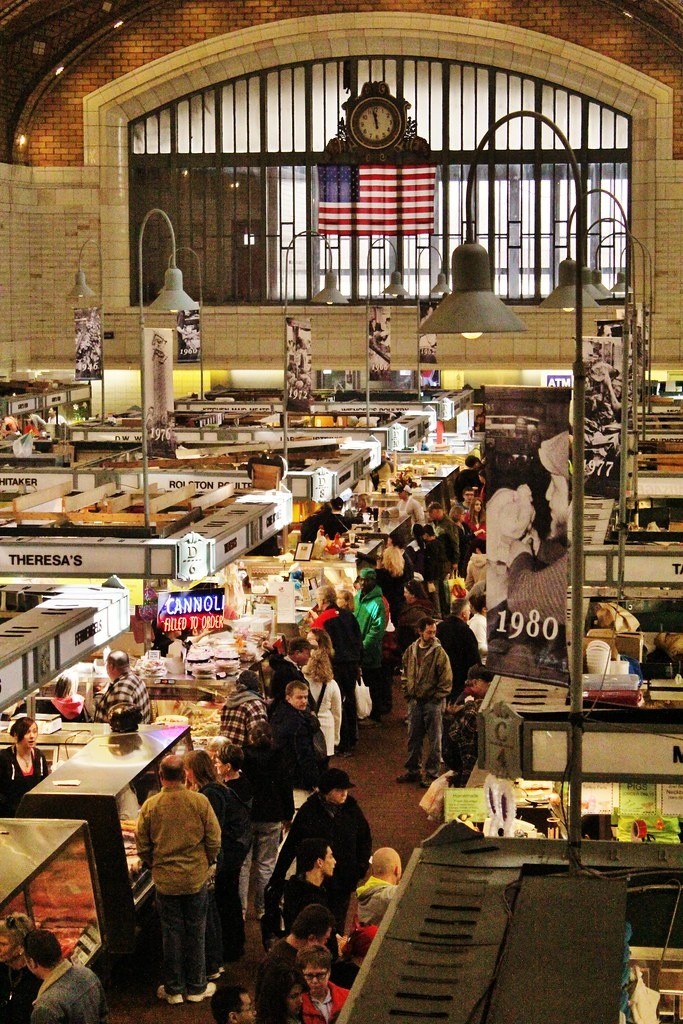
[355,676,372,718]
[441,568,467,603]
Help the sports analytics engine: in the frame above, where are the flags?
[318,165,434,235]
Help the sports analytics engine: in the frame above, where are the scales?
[7,713,62,735]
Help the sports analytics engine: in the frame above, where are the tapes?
[633,820,647,838]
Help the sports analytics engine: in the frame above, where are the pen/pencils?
[531,802,549,805]
[0,831,10,834]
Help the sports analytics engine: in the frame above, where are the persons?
[495,430,568,627]
[645,632,683,676]
[475,412,485,430]
[269,585,366,755]
[585,360,621,472]
[368,316,390,351]
[407,487,485,616]
[273,682,321,776]
[454,447,526,502]
[0,650,152,818]
[220,671,270,745]
[135,722,295,1001]
[395,592,493,785]
[353,535,416,728]
[0,910,110,1024]
[210,768,401,1023]
[245,537,282,556]
[0,407,67,438]
[299,483,426,543]
[420,306,436,355]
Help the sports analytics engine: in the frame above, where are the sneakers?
[157,985,182,1005]
[186,982,215,1001]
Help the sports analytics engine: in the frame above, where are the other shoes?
[397,772,421,783]
[419,774,439,787]
[206,967,225,980]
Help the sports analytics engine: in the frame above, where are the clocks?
[336,80,417,165]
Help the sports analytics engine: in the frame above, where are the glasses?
[6,916,18,930]
[302,969,328,982]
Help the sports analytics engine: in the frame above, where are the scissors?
[642,834,655,842]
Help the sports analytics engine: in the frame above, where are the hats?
[360,568,376,579]
[289,636,317,651]
[423,502,442,513]
[537,429,572,477]
[394,484,412,494]
[236,671,259,691]
[330,497,343,510]
[316,767,356,795]
